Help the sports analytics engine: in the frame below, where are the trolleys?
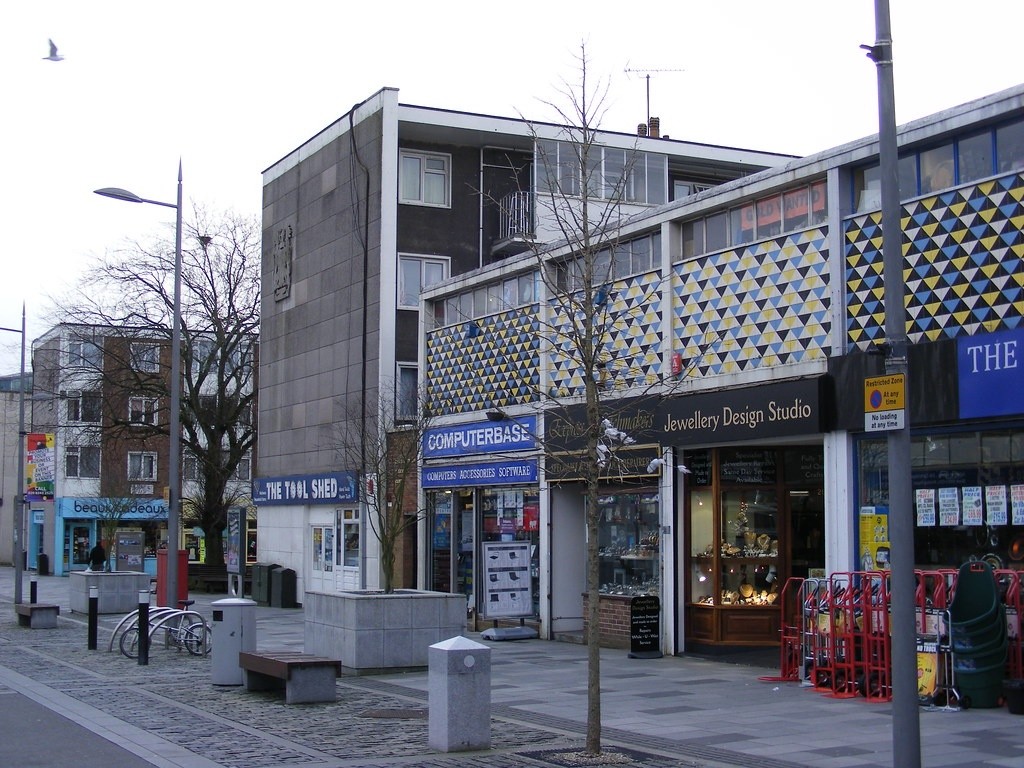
[758,567,1024,710]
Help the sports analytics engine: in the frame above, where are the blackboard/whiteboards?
[630,595,660,652]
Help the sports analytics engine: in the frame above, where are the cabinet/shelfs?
[685,447,791,647]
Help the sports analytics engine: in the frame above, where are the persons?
[88,539,106,571]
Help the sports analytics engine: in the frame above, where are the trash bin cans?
[209,597,258,686]
[37,553,49,575]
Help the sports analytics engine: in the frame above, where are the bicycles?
[119,601,212,659]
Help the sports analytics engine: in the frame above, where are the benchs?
[239,652,342,705]
[15,604,60,629]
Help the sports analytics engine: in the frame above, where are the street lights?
[93,152,186,650]
